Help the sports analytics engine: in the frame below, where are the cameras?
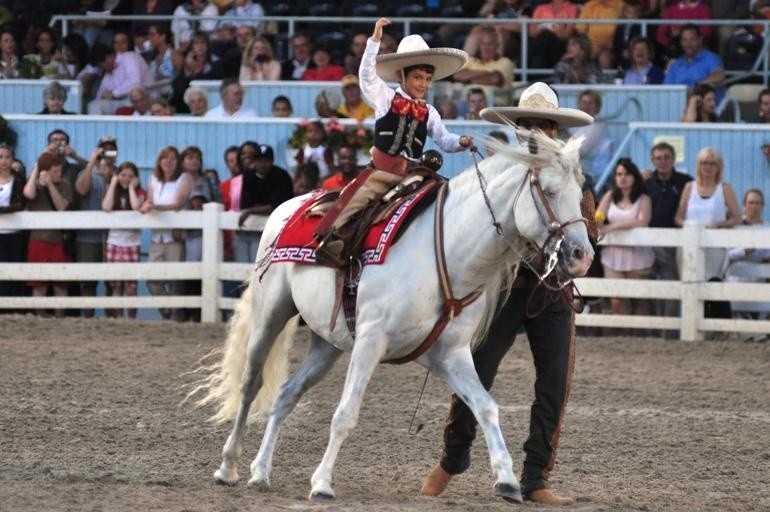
[56,140,66,148]
[97,150,117,161]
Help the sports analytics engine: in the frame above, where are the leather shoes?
[314,239,345,266]
[421,465,452,496]
[529,490,572,506]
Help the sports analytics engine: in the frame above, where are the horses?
[177,109,596,506]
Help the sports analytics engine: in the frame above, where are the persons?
[420,83,601,507]
[402,3,769,339]
[3,2,398,322]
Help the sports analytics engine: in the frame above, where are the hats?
[372,34,468,83]
[254,145,273,160]
[479,81,594,127]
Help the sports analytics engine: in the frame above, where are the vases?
[286,145,373,167]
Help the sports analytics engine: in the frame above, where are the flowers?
[288,117,374,155]
[21,54,41,79]
